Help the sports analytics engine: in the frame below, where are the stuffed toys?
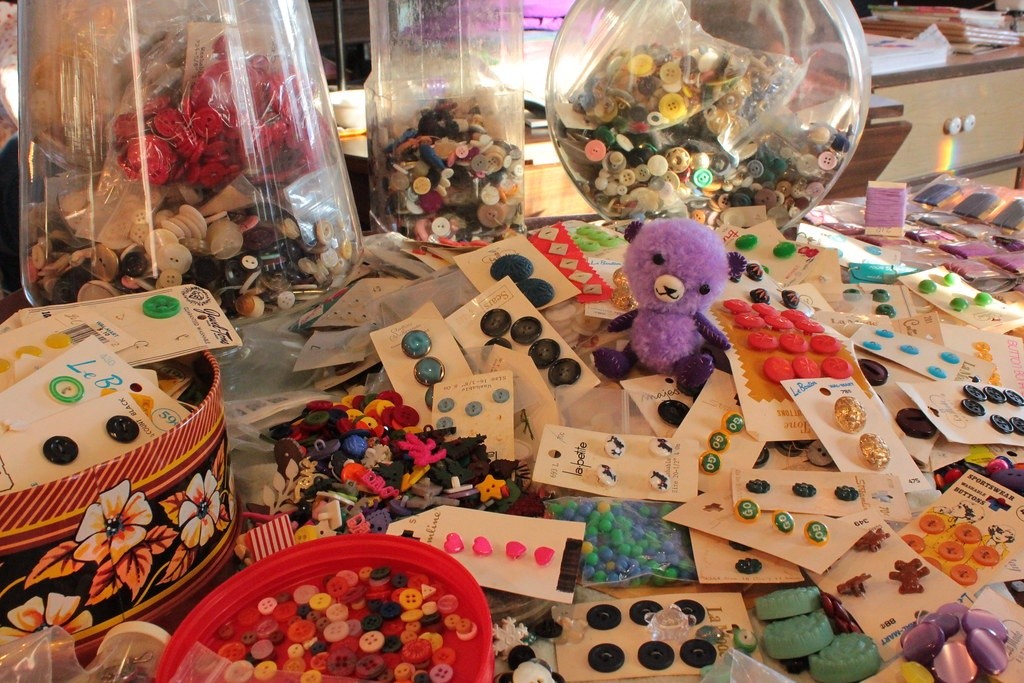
[592,217,746,392]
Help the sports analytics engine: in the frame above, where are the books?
[804,5,1020,79]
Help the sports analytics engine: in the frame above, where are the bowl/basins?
[329,90,366,128]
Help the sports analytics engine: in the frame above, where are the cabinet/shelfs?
[340,38,1024,218]
